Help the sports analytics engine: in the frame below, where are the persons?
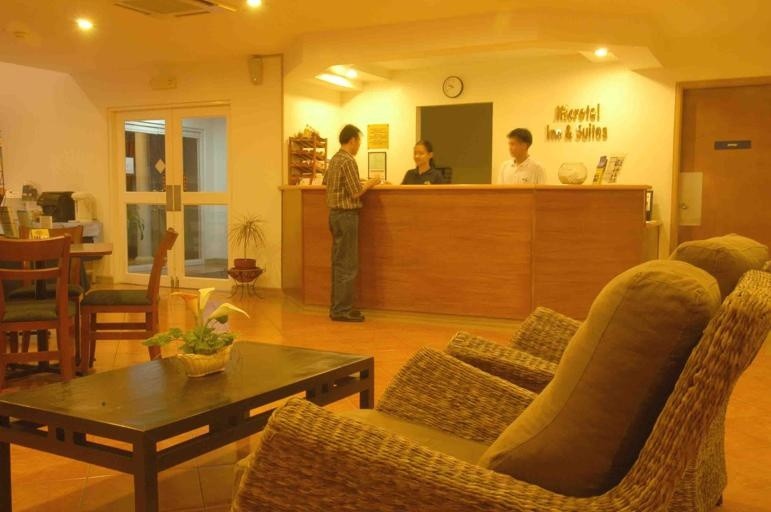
[399,139,447,184]
[497,128,548,184]
[321,125,381,322]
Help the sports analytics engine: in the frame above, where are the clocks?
[442,76,463,98]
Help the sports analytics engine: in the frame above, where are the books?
[0,206,32,238]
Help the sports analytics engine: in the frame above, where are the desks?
[2,241,112,380]
[27,222,83,285]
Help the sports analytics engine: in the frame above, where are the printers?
[38,190,97,223]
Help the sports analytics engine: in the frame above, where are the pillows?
[669,233,769,308]
[476,259,723,499]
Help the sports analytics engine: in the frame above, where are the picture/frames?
[6,198,37,238]
[368,152,386,181]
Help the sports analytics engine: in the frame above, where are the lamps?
[248,56,264,86]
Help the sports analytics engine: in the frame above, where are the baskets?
[159,331,235,378]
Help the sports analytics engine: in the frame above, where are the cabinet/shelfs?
[289,133,328,185]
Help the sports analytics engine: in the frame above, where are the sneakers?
[329,309,366,322]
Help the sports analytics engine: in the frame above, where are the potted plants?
[142,287,249,378]
[126,204,144,259]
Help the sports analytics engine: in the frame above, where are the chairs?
[230,259,771,511]
[77,228,179,373]
[445,233,771,393]
[0,233,77,382]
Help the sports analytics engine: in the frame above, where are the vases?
[226,213,263,268]
